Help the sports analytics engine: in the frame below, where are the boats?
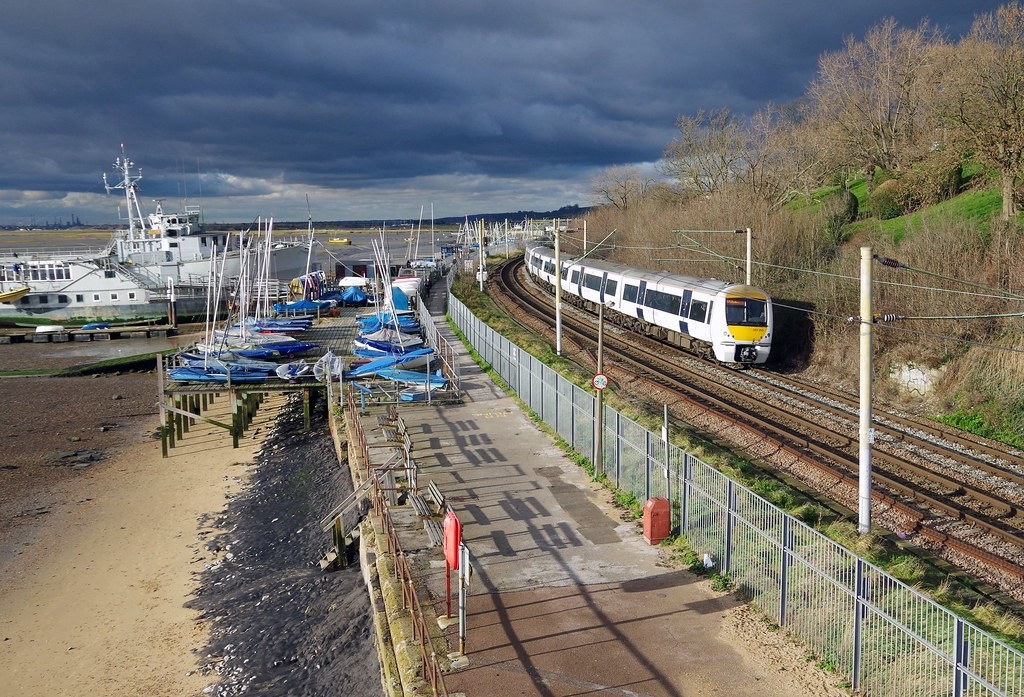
[329,232,352,245]
[0,144,320,328]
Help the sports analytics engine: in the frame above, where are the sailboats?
[166,214,341,384]
[337,203,519,401]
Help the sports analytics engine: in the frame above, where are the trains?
[524,236,773,363]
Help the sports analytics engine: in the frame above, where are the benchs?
[377,404,464,546]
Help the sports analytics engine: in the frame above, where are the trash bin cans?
[643,496,669,545]
